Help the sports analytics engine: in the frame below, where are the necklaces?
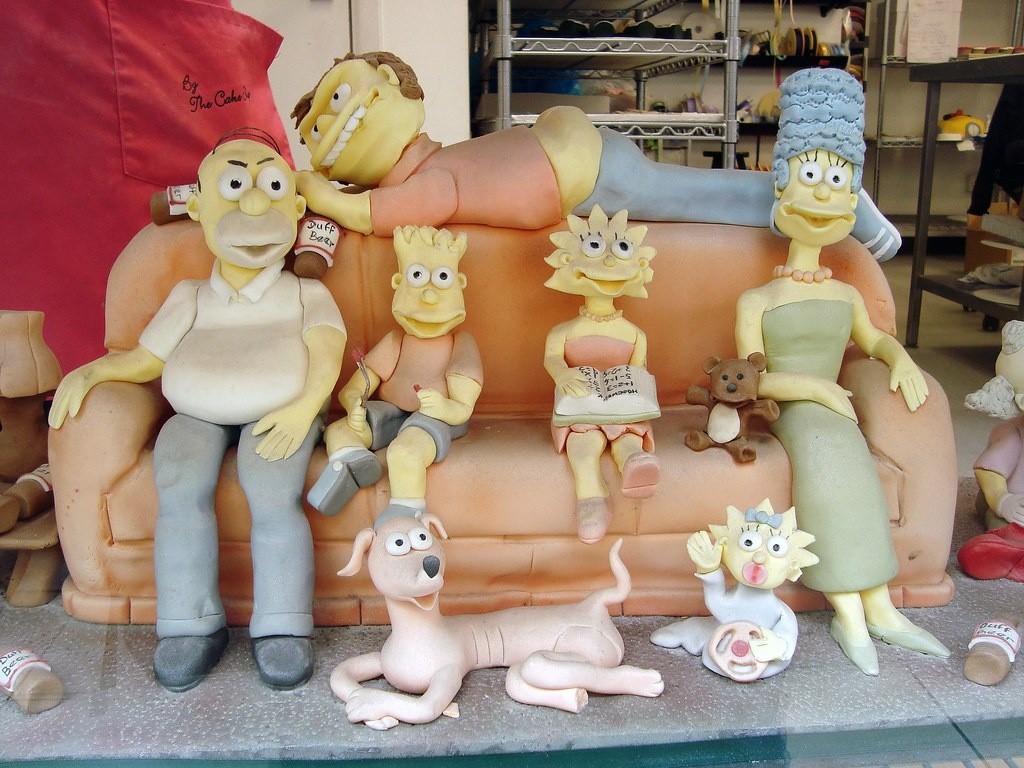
[773,265,833,283]
[579,305,624,322]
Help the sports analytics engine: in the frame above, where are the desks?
[903,53,1024,350]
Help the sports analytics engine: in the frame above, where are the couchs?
[46,218,961,627]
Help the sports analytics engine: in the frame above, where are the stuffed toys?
[685,352,780,463]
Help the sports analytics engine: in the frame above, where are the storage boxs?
[964,202,1024,275]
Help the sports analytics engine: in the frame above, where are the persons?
[290,52,902,261]
[957,320,1024,584]
[306,227,484,532]
[735,68,951,677]
[543,202,662,544]
[650,497,819,681]
[48,127,347,694]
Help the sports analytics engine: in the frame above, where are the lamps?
[0,309,62,481]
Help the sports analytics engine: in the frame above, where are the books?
[552,365,661,427]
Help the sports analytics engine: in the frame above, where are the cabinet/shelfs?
[872,0,1024,238]
[469,0,741,170]
[642,0,871,172]
